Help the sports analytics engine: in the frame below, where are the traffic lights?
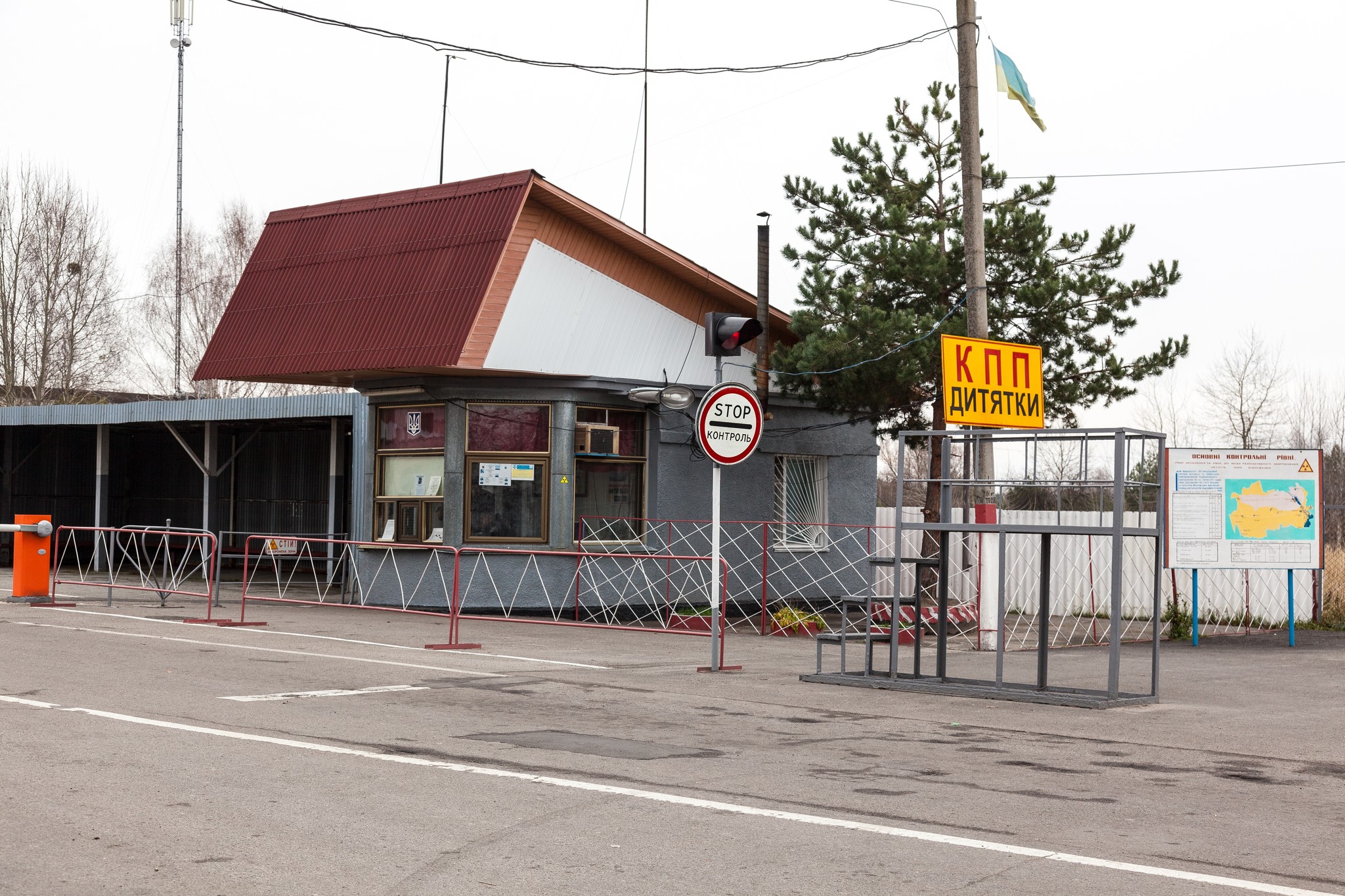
[702,312,766,359]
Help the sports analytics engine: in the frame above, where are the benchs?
[49,539,327,566]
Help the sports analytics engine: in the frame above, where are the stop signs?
[697,385,763,467]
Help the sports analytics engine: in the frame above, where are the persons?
[480,509,511,537]
[436,499,444,523]
[470,476,495,536]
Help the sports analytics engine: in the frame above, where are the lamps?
[607,387,695,410]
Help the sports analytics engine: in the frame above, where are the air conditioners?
[575,424,619,455]
